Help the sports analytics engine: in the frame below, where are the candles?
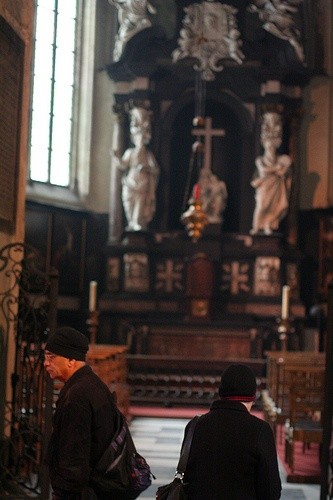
[281,286,291,319]
[88,280,98,312]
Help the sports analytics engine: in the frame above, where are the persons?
[177,365,282,500]
[249,111,291,236]
[114,107,162,233]
[249,0,304,63]
[110,0,157,62]
[43,326,136,500]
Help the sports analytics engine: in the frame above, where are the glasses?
[45,353,58,363]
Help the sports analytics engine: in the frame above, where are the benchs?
[259,350,325,472]
[20,340,128,422]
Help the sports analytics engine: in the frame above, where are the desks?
[125,352,267,408]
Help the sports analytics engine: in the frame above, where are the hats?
[45,326,88,362]
[218,364,257,401]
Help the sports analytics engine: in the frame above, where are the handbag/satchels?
[89,416,156,500]
[154,476,189,500]
